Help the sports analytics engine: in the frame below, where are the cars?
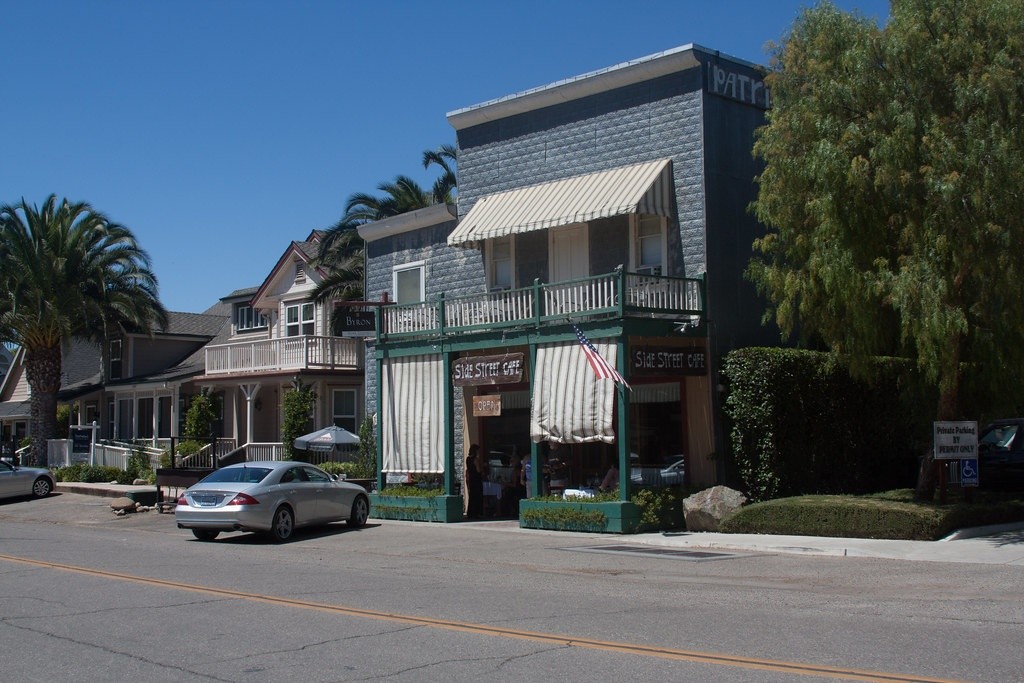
[175,461,370,543]
[0,460,56,501]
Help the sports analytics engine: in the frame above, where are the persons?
[466,443,486,519]
[520,452,548,498]
[547,440,574,481]
[599,459,619,492]
[501,455,520,516]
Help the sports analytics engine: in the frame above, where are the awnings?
[446,159,674,249]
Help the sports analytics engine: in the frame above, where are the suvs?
[978,418,1024,494]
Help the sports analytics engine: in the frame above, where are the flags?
[571,322,633,391]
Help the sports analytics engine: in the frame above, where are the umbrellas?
[293,423,360,473]
